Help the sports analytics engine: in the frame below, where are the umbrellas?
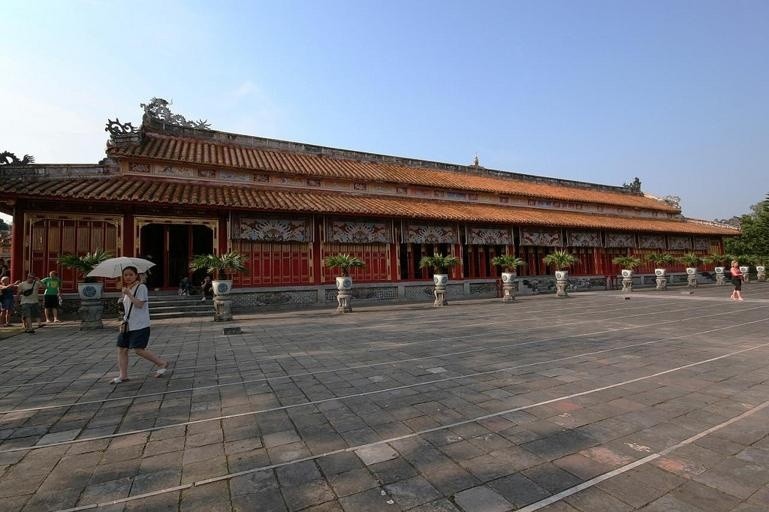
[87,256,157,287]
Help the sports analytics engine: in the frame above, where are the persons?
[17,272,48,334]
[40,271,62,323]
[109,265,170,384]
[730,260,744,302]
[199,275,213,302]
[178,276,191,296]
[0,276,20,326]
[0,258,10,278]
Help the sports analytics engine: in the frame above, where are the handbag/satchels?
[119,322,129,337]
[24,289,33,296]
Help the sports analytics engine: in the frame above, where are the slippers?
[110,377,129,384]
[156,362,169,378]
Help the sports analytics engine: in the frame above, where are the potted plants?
[737,254,751,273]
[711,253,729,274]
[543,247,580,280]
[645,251,674,277]
[489,254,529,284]
[56,245,114,302]
[752,255,769,272]
[611,255,643,280]
[322,252,367,291]
[678,250,702,274]
[186,248,247,294]
[418,251,463,290]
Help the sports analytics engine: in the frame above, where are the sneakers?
[201,297,207,302]
